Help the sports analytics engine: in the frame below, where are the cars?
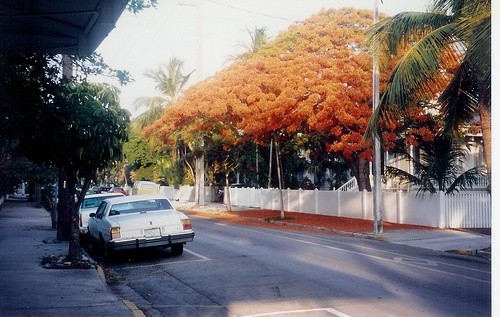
[77,193,134,233]
[87,194,195,265]
